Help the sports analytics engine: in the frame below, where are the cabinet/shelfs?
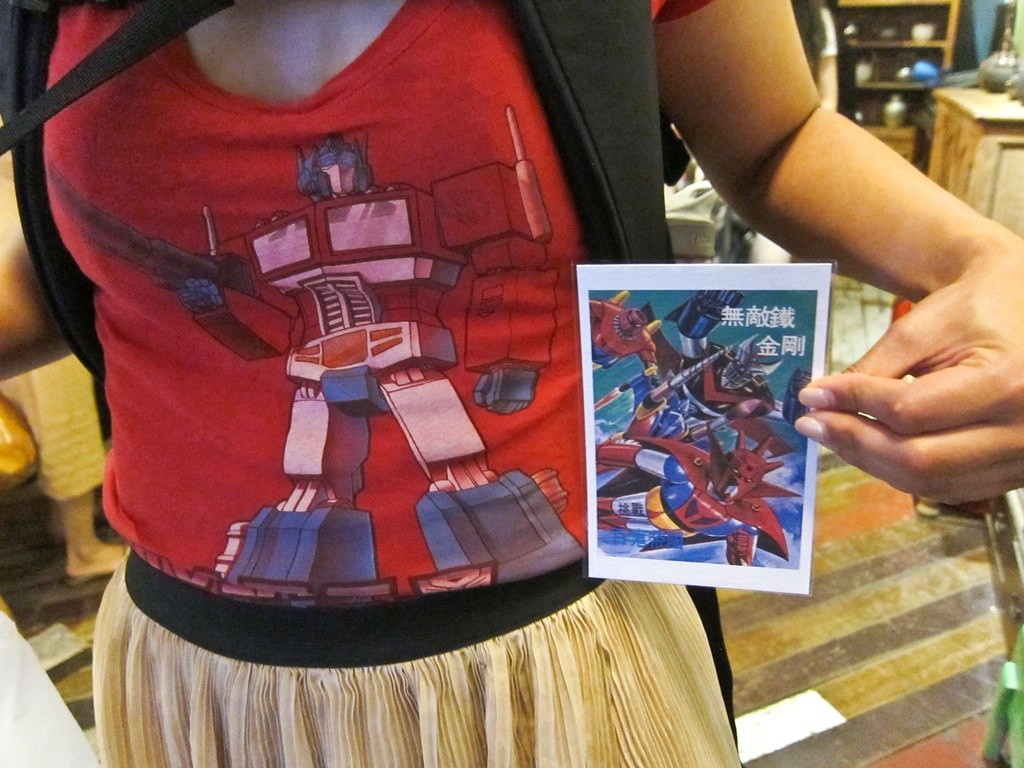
[836,0,1024,244]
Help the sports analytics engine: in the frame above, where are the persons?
[1,0,1024,768]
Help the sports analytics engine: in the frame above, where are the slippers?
[64,544,130,586]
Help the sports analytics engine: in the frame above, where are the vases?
[978,0,1024,94]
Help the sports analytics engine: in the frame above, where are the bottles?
[911,22,933,41]
[883,93,910,128]
